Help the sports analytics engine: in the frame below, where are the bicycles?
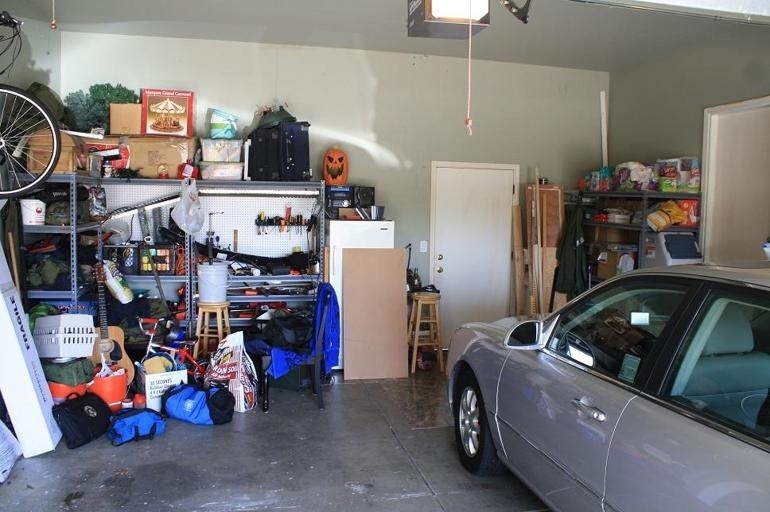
[0,9,61,199]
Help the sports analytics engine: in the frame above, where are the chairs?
[685,303,770,431]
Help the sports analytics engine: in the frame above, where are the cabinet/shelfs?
[575,191,703,290]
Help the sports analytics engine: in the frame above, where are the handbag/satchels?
[267,309,313,351]
[106,407,166,444]
[53,389,114,449]
[203,331,258,412]
[162,380,235,425]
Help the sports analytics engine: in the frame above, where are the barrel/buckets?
[197,260,231,305]
[88,365,129,414]
[48,382,88,405]
[19,197,46,226]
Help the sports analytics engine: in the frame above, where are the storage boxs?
[135,365,190,412]
[79,89,201,178]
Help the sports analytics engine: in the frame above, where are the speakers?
[354,185,375,207]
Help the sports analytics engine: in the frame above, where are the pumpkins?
[577,175,586,192]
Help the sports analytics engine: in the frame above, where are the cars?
[444,264,769,512]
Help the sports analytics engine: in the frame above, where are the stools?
[408,292,445,375]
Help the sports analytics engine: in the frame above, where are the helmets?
[164,327,185,348]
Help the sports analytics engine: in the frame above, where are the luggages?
[248,121,311,182]
